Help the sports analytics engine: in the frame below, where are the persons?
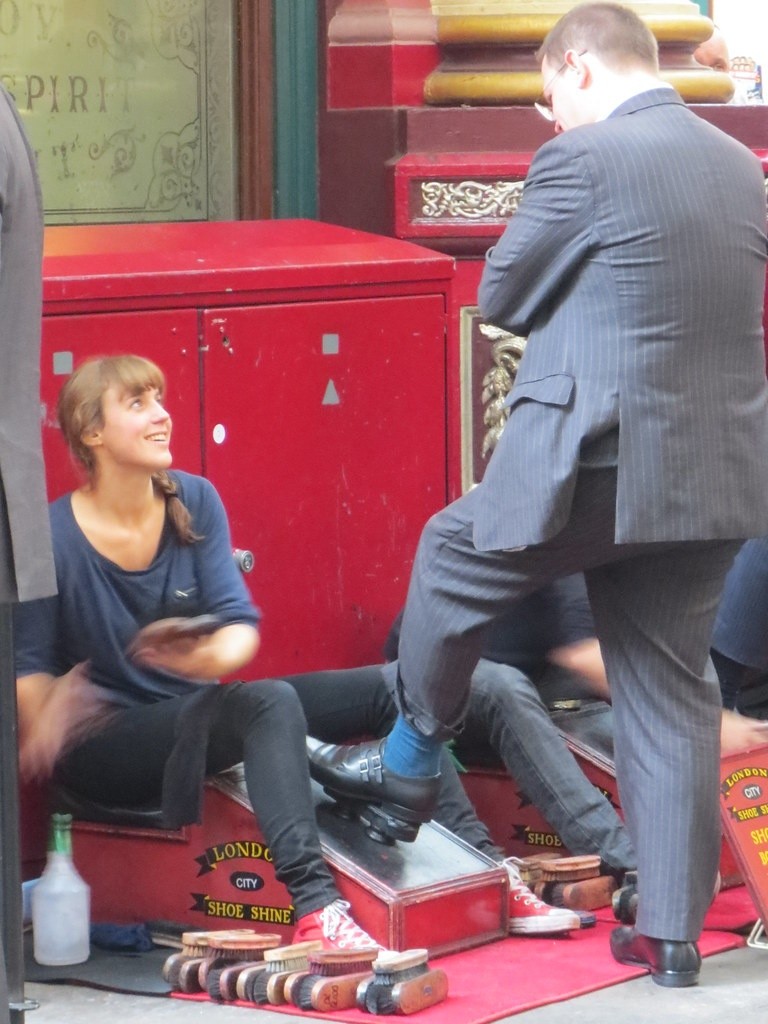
[304,1,768,988]
[0,80,59,1022]
[16,353,582,967]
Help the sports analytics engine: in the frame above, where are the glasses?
[534,49,589,121]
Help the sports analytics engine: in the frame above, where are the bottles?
[31,814,91,966]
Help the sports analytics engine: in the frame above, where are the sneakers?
[495,857,580,934]
[291,899,401,961]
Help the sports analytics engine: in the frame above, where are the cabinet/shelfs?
[40,217,457,688]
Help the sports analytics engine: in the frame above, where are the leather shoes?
[611,926,702,987]
[305,735,443,824]
